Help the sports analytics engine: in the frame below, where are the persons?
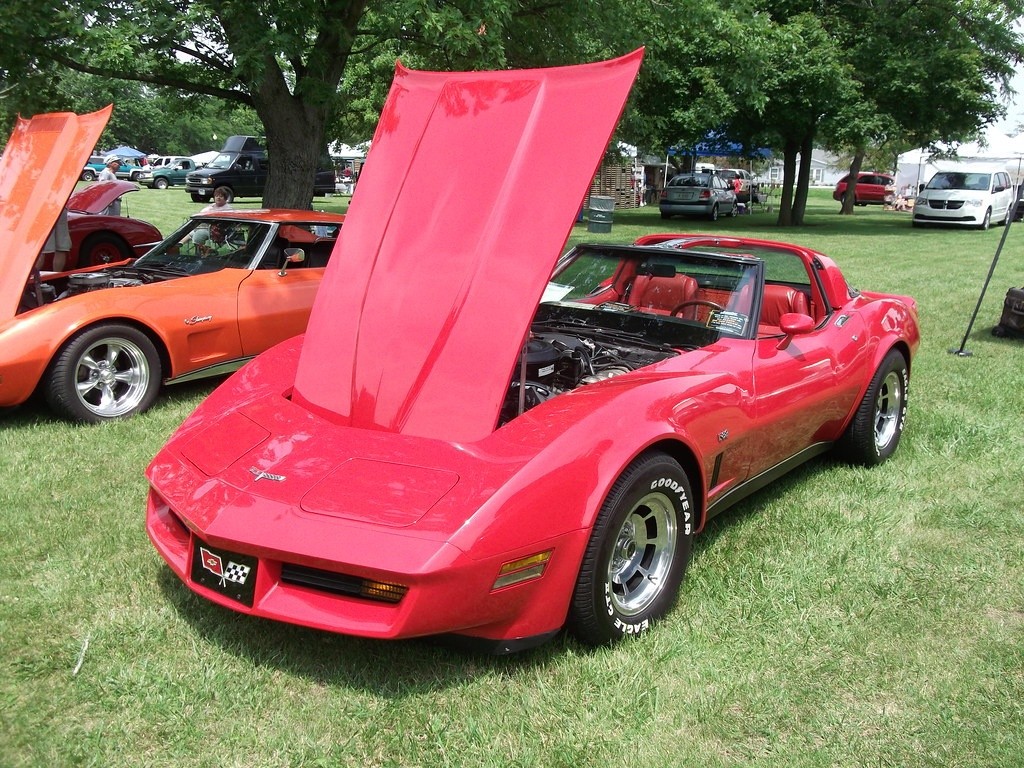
[98,154,122,215]
[343,166,352,178]
[884,181,907,211]
[35,208,73,272]
[175,187,234,250]
[733,174,743,193]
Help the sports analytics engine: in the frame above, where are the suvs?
[142,156,193,170]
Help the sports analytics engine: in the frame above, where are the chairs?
[725,284,811,338]
[185,218,230,257]
[300,240,336,268]
[627,272,698,323]
[230,236,289,271]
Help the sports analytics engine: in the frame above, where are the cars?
[832,171,896,206]
[659,174,739,221]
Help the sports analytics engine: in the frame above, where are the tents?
[664,129,774,213]
[101,146,146,166]
[328,138,371,177]
[618,142,637,208]
[894,127,1024,207]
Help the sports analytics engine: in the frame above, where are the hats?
[103,154,120,167]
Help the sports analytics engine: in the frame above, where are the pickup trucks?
[137,159,207,189]
[81,158,143,182]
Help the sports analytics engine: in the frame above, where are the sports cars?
[141,44,924,650]
[43,179,162,272]
[1,103,348,426]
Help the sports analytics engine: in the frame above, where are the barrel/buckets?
[588,195,615,234]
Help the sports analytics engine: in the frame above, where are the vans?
[185,136,335,203]
[714,168,752,192]
[912,166,1014,231]
